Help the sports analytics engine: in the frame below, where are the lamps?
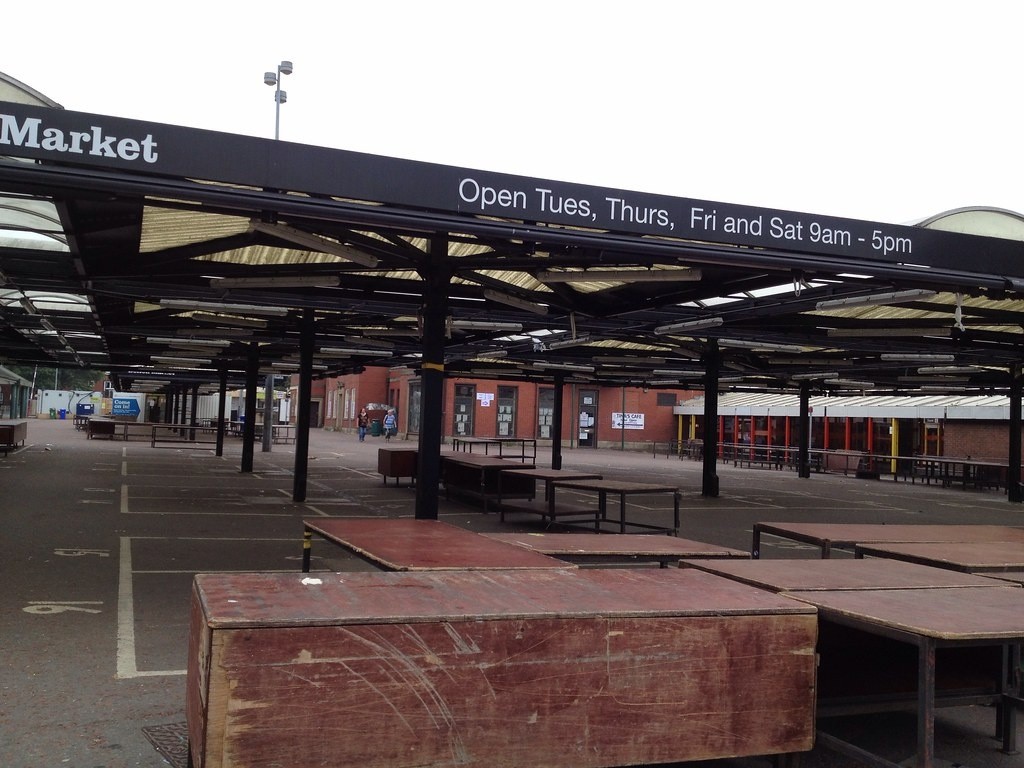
[655,317,724,335]
[20,218,549,392]
[717,339,802,354]
[790,372,840,381]
[672,347,700,359]
[653,370,705,377]
[533,362,594,373]
[918,367,982,374]
[897,376,970,382]
[536,268,703,283]
[816,289,936,312]
[920,386,966,392]
[823,379,874,389]
[767,358,854,366]
[549,337,593,350]
[718,377,744,382]
[880,354,955,361]
[827,328,952,337]
[592,357,665,364]
[597,371,654,377]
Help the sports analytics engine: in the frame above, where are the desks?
[0,414,1024,768]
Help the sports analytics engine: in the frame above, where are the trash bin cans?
[50,408,56,419]
[371,419,380,436]
[59,408,66,419]
[239,415,245,421]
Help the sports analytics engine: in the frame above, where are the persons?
[383,410,397,443]
[357,408,369,442]
